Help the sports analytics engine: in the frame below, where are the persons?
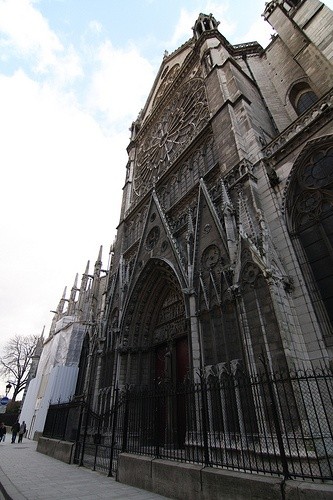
[17,421,26,444]
[10,419,20,444]
[0,423,6,443]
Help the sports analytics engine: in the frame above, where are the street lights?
[2,382,12,416]
